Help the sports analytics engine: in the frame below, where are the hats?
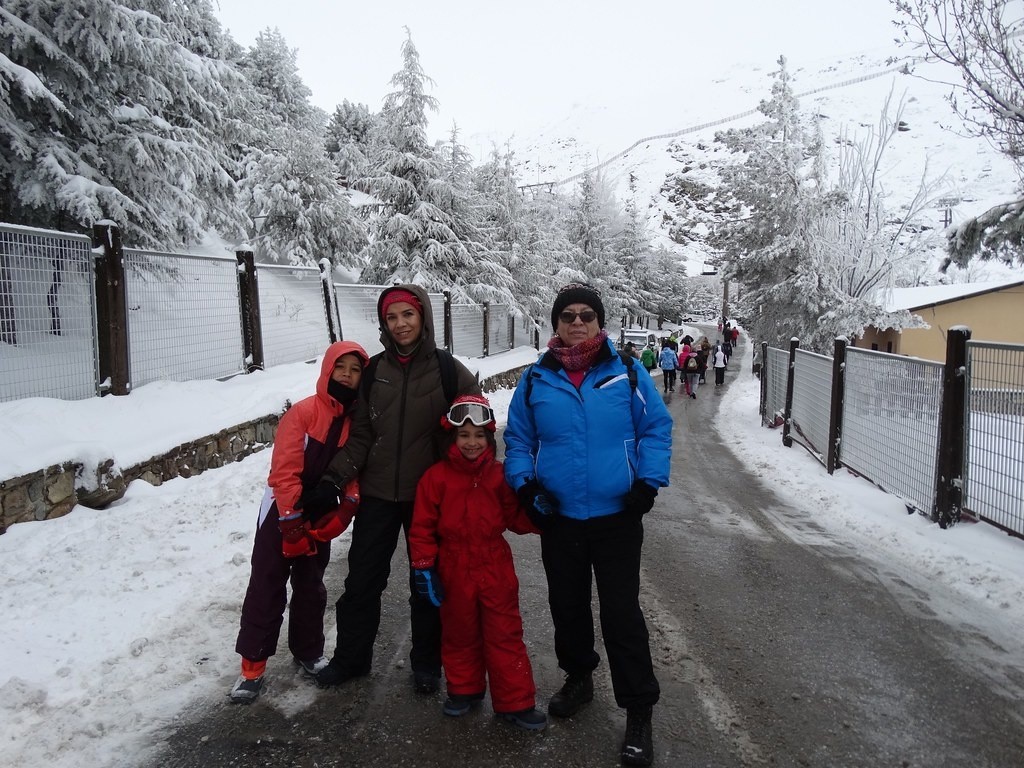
[551,282,604,333]
[382,289,425,322]
[440,393,496,434]
[352,352,365,368]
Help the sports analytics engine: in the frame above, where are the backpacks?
[687,357,700,370]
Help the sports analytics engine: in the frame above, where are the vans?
[681,310,707,323]
[615,329,659,363]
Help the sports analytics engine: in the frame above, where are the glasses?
[446,402,495,427]
[560,311,598,324]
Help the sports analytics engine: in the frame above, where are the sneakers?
[444,691,487,715]
[620,705,655,768]
[549,672,596,717]
[502,705,547,729]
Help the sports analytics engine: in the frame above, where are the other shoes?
[662,374,723,399]
[293,653,330,674]
[314,661,362,688]
[413,661,440,693]
[228,673,265,704]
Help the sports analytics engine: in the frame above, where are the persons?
[623,341,640,360]
[230,341,369,703]
[313,284,482,688]
[718,315,739,347]
[503,281,674,768]
[639,339,660,374]
[701,336,732,387]
[659,335,680,393]
[408,395,547,729]
[677,334,708,399]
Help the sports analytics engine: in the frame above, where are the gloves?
[277,513,318,558]
[517,478,566,534]
[414,567,446,608]
[305,497,359,542]
[623,480,661,516]
[293,480,343,528]
